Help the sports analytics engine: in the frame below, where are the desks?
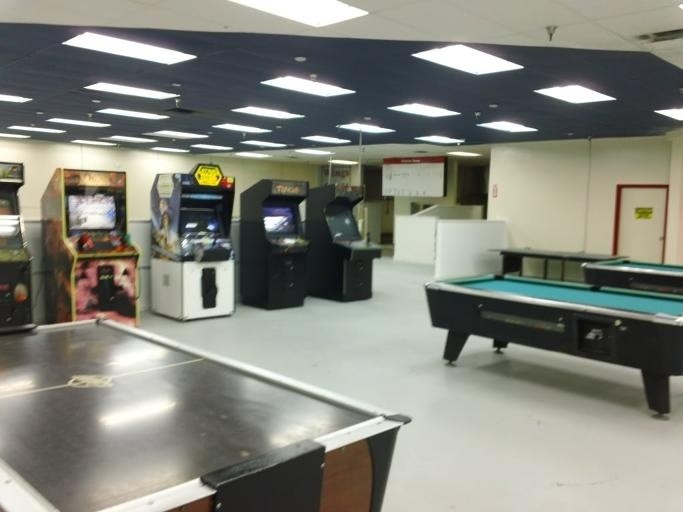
[0,317,410,512]
[426,274,683,414]
[490,248,625,286]
[580,260,683,296]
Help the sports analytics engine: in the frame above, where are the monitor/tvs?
[261,205,299,238]
[0,198,22,249]
[325,205,361,241]
[178,209,222,240]
[67,194,117,231]
